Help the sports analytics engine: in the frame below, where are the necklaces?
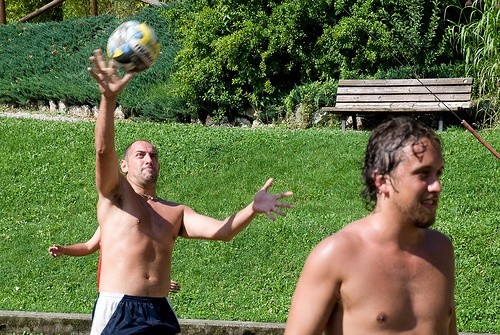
[138,192,158,200]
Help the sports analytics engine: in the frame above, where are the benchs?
[322,78,475,132]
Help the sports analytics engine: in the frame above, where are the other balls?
[107,20,160,73]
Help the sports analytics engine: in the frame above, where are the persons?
[49,224,180,294]
[283,116,458,335]
[85,48,293,335]
